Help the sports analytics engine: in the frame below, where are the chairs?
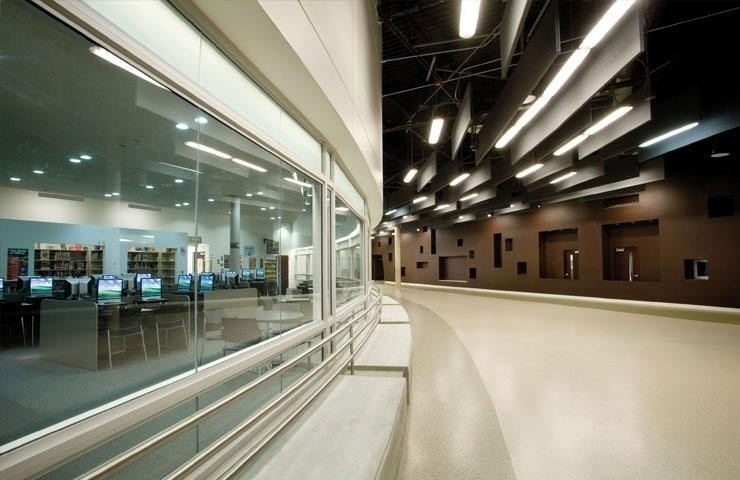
[198,300,317,372]
[5,284,196,371]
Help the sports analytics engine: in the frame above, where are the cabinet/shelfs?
[128,249,176,282]
[34,246,104,281]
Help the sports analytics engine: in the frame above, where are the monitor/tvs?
[0,277,4,292]
[224,272,240,287]
[64,273,152,299]
[177,275,192,291]
[18,276,41,293]
[255,267,266,280]
[96,279,123,305]
[241,268,252,281]
[199,273,213,292]
[140,278,163,301]
[29,276,53,296]
